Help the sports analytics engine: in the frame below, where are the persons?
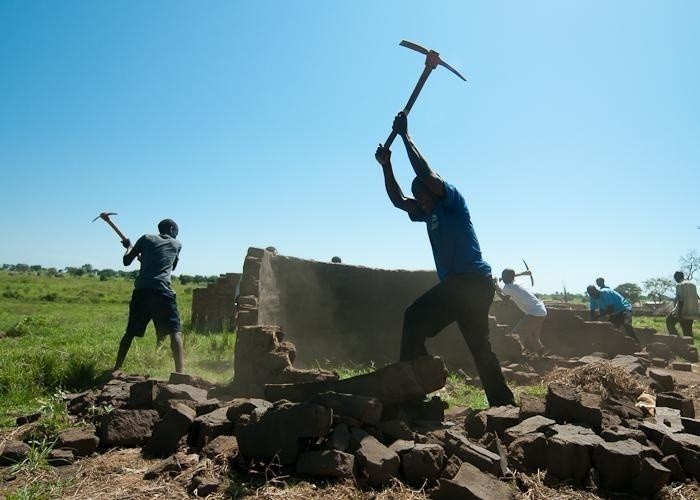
[665,271,700,337]
[587,284,639,342]
[494,269,547,355]
[375,111,516,407]
[332,255,341,263]
[114,219,185,375]
[596,278,609,289]
[235,246,278,299]
